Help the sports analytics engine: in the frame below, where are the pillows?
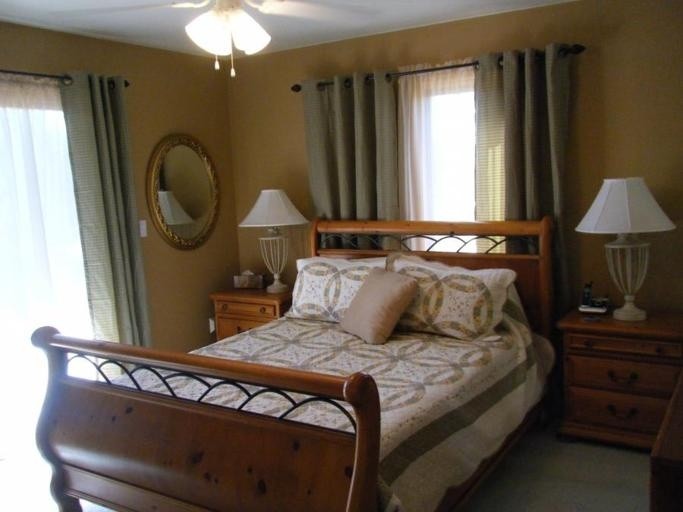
[283,251,519,346]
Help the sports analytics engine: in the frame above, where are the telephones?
[577,281,611,314]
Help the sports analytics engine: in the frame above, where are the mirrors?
[145,133,222,251]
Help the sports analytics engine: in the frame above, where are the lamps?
[236,187,311,293]
[572,176,678,323]
[183,0,271,77]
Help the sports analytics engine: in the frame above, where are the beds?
[29,217,557,512]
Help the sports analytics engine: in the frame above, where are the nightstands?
[207,289,293,342]
[557,301,683,454]
